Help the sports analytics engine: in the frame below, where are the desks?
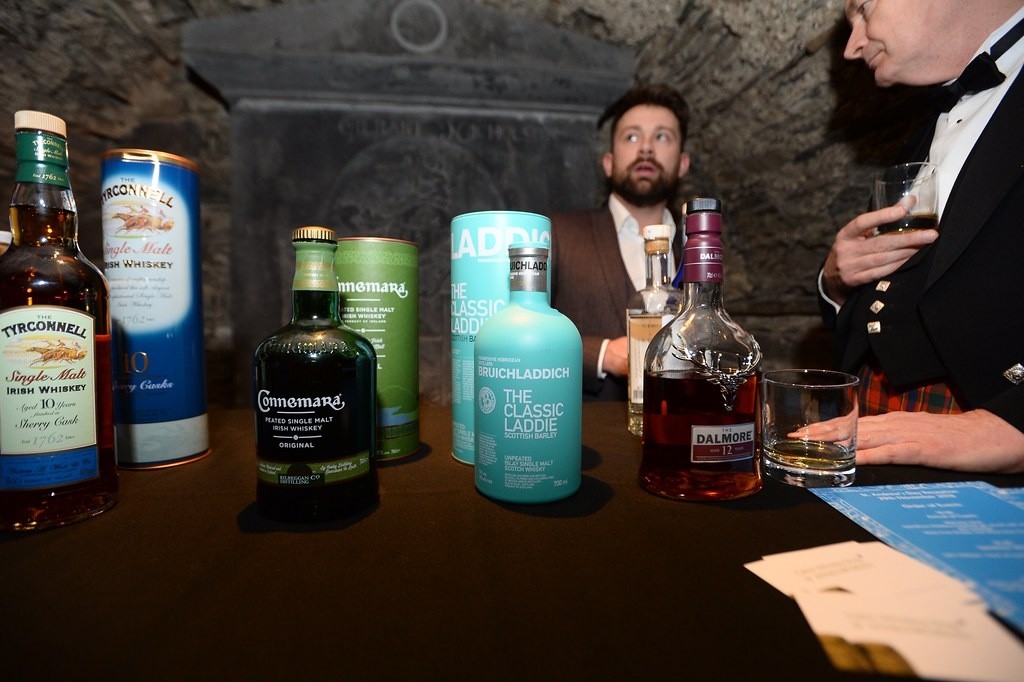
[0,402,1024,682]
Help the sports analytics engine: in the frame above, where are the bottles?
[638,197,762,503]
[0,110,117,535]
[473,241,584,505]
[253,225,379,525]
[625,224,683,438]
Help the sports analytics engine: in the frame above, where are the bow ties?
[933,51,1006,114]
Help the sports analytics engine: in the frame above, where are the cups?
[761,369,861,488]
[870,161,939,235]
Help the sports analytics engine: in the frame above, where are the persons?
[550,82,690,402]
[787,0,1024,474]
